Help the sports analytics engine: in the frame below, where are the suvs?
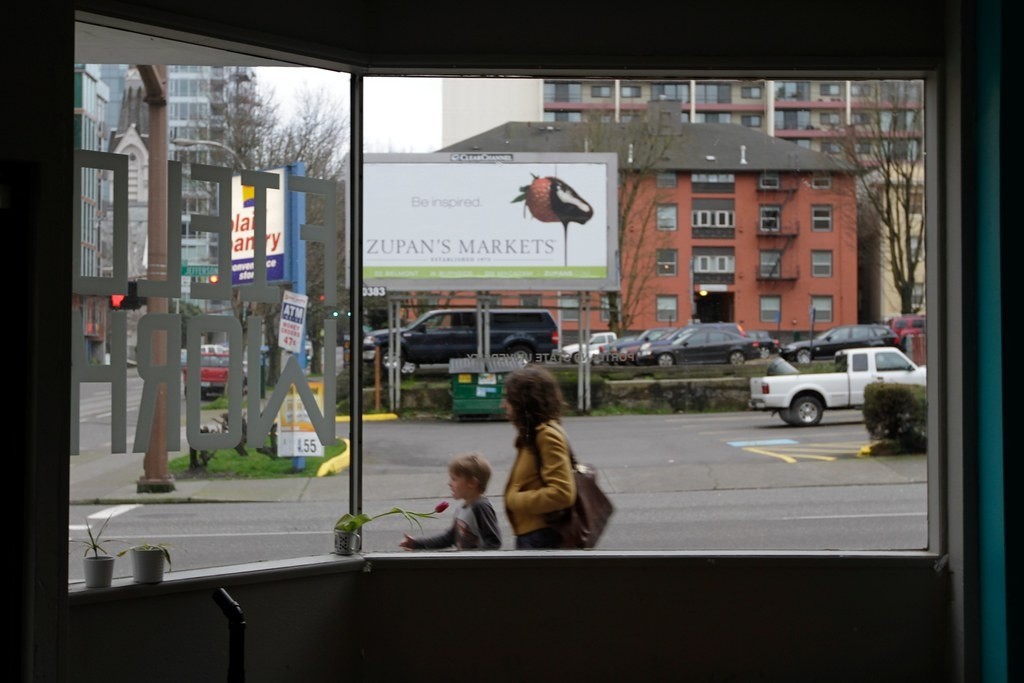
[636,323,747,355]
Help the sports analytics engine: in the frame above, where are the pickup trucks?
[748,347,927,428]
[562,331,618,365]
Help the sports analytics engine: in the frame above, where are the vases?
[333,528,362,556]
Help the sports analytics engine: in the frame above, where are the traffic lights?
[110,294,141,311]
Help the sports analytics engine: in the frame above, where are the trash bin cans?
[448,356,525,415]
[766,357,799,374]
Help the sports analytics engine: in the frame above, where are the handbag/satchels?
[532,421,613,550]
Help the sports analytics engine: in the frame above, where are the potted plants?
[69,514,131,589]
[116,541,174,584]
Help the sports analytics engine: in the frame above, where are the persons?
[501,368,577,549]
[400,453,501,551]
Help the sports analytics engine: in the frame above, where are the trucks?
[182,352,231,400]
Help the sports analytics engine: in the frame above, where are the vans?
[362,308,558,374]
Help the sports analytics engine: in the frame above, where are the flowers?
[334,500,450,534]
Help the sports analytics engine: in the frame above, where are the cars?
[599,330,676,366]
[748,331,780,354]
[636,329,760,367]
[779,324,901,363]
[886,316,926,345]
